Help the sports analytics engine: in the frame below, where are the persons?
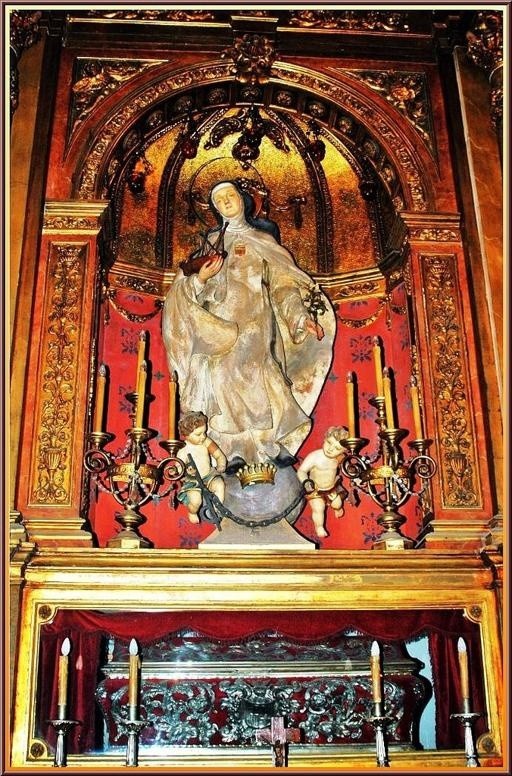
[162,178,327,476]
[167,408,230,525]
[292,425,360,539]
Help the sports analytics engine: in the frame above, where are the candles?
[169,382,177,440]
[93,377,106,432]
[410,386,422,439]
[368,641,384,707]
[347,382,355,438]
[457,637,470,702]
[373,346,384,396]
[136,371,147,428]
[55,638,71,704]
[136,341,146,393]
[383,377,395,429]
[128,639,141,707]
